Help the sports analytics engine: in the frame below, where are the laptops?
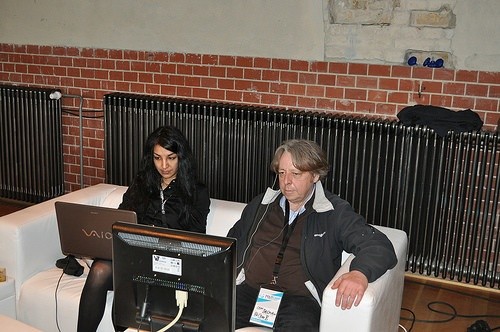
[55,201,138,261]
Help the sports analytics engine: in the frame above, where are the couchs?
[0,182,407,332]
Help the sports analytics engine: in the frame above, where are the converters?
[467,320,492,332]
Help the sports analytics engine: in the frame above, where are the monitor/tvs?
[112,222,238,332]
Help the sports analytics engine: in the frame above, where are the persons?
[77,126,210,332]
[226,139,397,332]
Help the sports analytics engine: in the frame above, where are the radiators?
[103,91,500,289]
[0,84,84,203]
